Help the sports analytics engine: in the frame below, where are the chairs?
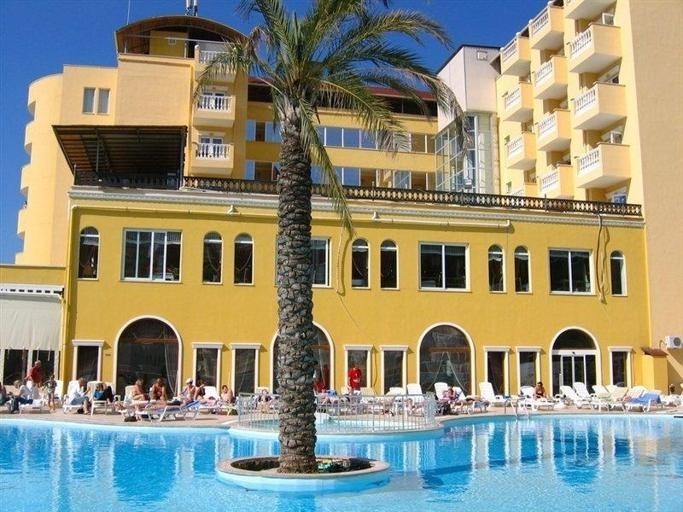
[0,360,681,427]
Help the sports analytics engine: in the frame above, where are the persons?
[0,374,237,422]
[313,362,363,405]
[448,384,456,409]
[258,389,271,403]
[533,382,547,401]
[29,360,43,388]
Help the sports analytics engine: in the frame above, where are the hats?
[186,378,192,384]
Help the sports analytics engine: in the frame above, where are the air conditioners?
[665,335,682,349]
